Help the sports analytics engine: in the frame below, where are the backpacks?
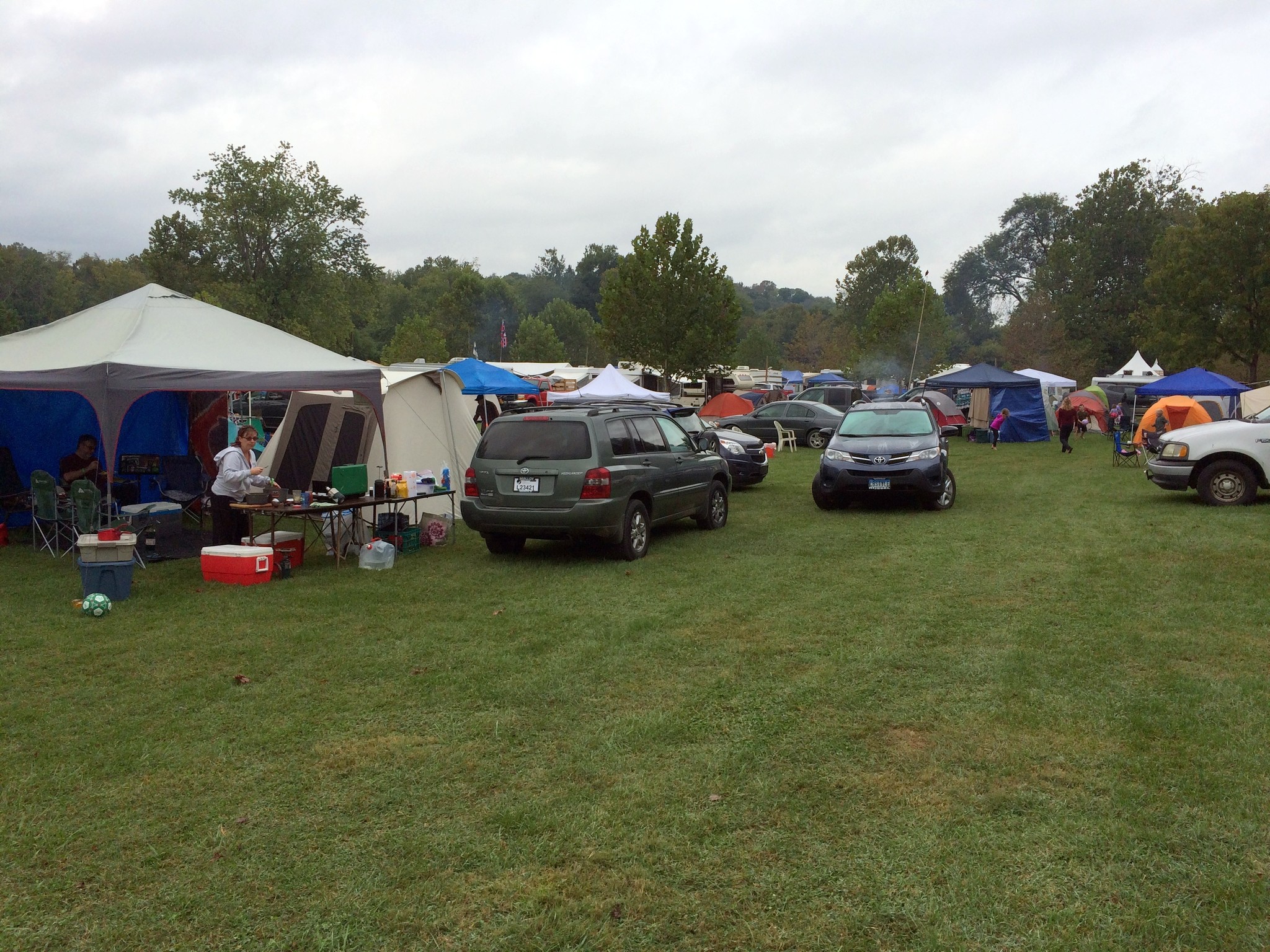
[1111,413,1118,417]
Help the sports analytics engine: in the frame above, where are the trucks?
[640,366,867,408]
[498,377,577,413]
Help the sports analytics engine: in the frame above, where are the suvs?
[812,398,955,511]
[460,405,732,561]
[1145,406,1270,506]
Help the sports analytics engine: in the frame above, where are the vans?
[1092,375,1167,416]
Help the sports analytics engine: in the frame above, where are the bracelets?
[82,468,85,475]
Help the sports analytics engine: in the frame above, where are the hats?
[708,395,712,398]
[474,394,483,400]
[528,398,536,400]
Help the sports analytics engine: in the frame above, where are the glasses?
[708,398,711,398]
[527,400,535,402]
[85,445,97,450]
[241,436,258,441]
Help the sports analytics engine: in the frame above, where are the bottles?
[395,480,408,498]
[390,472,403,480]
[442,469,451,490]
[325,486,345,504]
[369,487,373,497]
[390,480,397,498]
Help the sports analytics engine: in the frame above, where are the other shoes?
[991,444,993,448]
[1062,447,1067,452]
[1074,438,1077,441]
[1080,437,1085,439]
[1068,448,1073,453]
[993,448,997,450]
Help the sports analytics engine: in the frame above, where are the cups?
[403,471,417,497]
[300,492,310,507]
[1144,433,1147,437]
[305,491,313,505]
[292,490,302,505]
[375,479,389,499]
[1128,441,1132,444]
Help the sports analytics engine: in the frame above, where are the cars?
[714,381,970,449]
[546,399,769,490]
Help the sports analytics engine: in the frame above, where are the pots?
[97,523,133,541]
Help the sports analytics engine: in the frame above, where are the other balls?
[1082,419,1089,425]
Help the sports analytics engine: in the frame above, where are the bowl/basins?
[263,488,289,503]
[245,493,270,504]
[416,482,435,495]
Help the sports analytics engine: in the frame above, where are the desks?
[230,493,392,568]
[351,490,456,545]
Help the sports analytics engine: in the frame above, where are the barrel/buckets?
[358,537,395,571]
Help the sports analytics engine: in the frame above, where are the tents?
[0,282,1270,546]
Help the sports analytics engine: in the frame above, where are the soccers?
[83,593,112,617]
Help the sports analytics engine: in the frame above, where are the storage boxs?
[374,528,423,554]
[200,544,273,585]
[311,459,369,498]
[77,532,137,564]
[240,531,306,572]
[120,501,182,517]
[962,425,1000,444]
[78,557,136,602]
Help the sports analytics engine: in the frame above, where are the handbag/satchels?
[378,512,410,532]
[967,431,976,442]
[420,512,448,547]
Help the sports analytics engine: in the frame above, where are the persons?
[1057,397,1077,453]
[58,434,115,498]
[473,394,500,436]
[1109,404,1119,416]
[210,425,281,545]
[1151,409,1169,433]
[1075,404,1089,439]
[990,408,1009,450]
[1116,403,1123,416]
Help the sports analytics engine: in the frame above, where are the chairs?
[0,447,38,524]
[1107,416,1130,441]
[1113,430,1140,469]
[31,470,75,560]
[152,454,205,523]
[774,421,797,453]
[1141,428,1167,465]
[71,479,101,567]
[732,426,743,433]
